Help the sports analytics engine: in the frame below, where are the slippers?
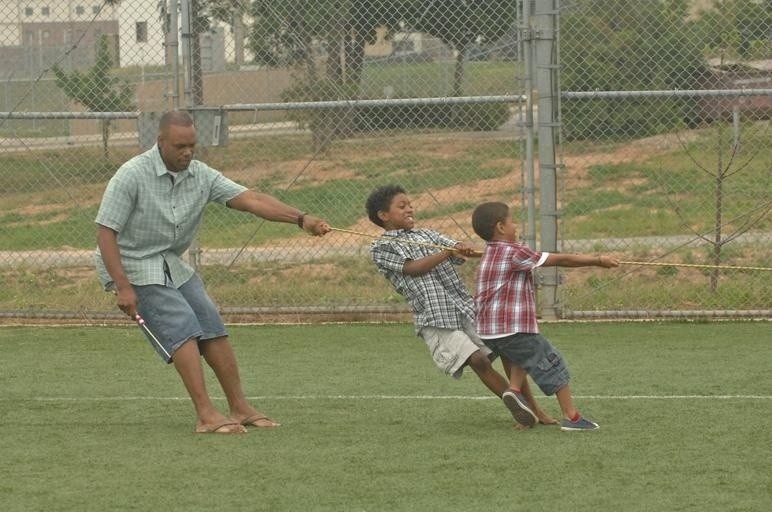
[240,413,281,428]
[206,417,247,433]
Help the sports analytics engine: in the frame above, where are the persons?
[365,184,560,427]
[471,202,621,432]
[95,108,333,437]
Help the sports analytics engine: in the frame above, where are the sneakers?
[502,388,538,427]
[560,415,600,431]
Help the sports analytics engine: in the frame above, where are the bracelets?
[297,211,310,229]
